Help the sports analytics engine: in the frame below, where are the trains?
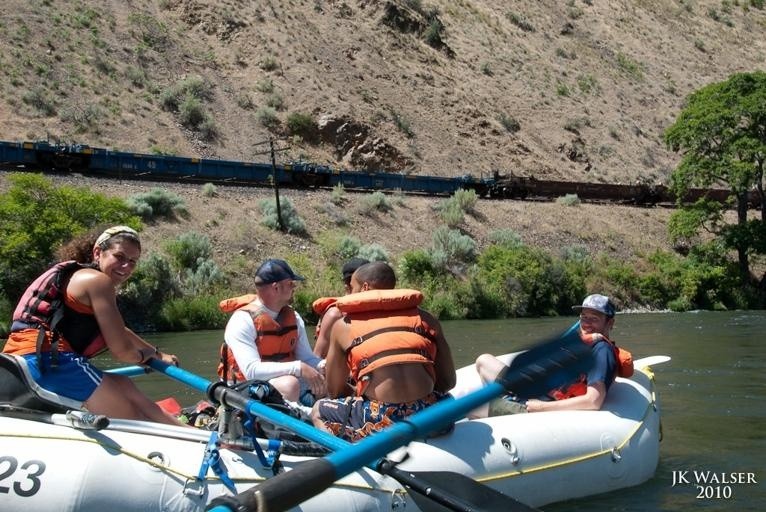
[0,140,766,210]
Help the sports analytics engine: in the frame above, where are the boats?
[0,350,663,512]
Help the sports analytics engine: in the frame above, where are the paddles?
[148,358,537,511]
[147,330,598,512]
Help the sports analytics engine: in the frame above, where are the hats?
[571,293,616,319]
[253,258,306,286]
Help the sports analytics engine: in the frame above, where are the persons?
[467,293,634,420]
[302,258,456,443]
[218,258,327,402]
[2,225,194,429]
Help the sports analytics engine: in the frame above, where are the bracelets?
[155,346,159,355]
[138,350,145,363]
